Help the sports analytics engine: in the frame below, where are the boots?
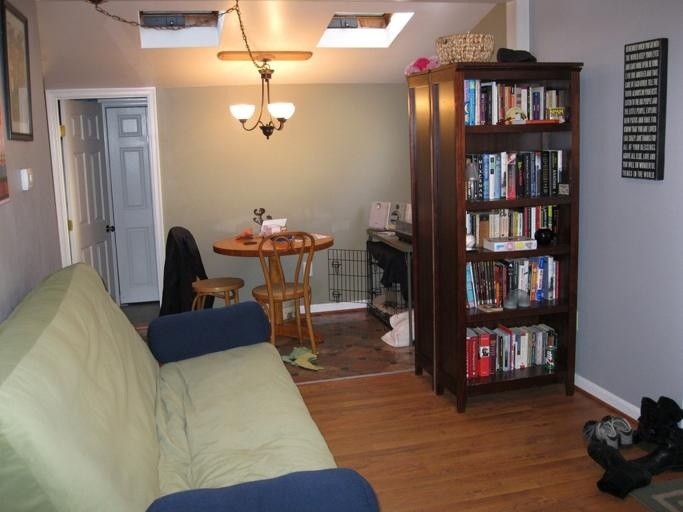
[635,394,682,444]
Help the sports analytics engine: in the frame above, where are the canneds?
[544,345,559,370]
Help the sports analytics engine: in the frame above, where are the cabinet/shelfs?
[403,68,442,395]
[425,60,586,413]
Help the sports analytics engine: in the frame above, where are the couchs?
[0,261,382,512]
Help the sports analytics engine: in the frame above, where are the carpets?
[627,474,683,512]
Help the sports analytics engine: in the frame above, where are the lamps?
[227,56,295,141]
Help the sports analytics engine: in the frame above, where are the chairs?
[250,230,320,356]
[164,226,245,312]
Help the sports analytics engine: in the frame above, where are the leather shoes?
[589,443,652,498]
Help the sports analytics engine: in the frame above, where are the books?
[462,79,568,379]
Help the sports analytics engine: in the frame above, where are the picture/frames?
[0,1,33,145]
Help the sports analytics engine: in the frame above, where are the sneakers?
[601,414,633,449]
[581,419,619,450]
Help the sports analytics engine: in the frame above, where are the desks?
[211,232,335,344]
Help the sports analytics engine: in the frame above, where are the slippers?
[630,430,682,474]
